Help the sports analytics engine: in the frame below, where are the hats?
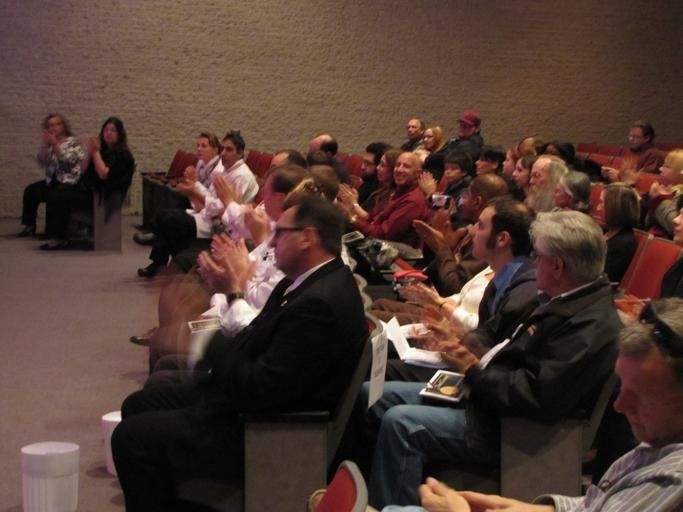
[456,110,481,126]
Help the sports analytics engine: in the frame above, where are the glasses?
[636,301,682,355]
[274,226,302,238]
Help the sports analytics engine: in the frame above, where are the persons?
[39,116,136,251]
[110,110,682,511]
[14,113,86,241]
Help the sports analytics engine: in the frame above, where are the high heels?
[17,223,35,237]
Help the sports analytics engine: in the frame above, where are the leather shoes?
[40,243,60,250]
[134,233,155,245]
[139,262,168,277]
[130,332,154,345]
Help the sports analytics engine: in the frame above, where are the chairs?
[47,147,683,512]
